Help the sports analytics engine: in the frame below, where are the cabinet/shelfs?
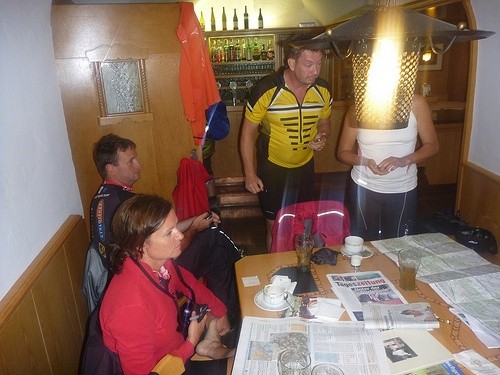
[205,34,279,104]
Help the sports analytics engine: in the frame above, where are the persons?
[90,133,231,360]
[337,93,439,242]
[99,194,230,375]
[371,294,384,301]
[402,307,437,321]
[239,37,332,253]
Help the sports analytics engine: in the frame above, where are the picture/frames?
[417,44,444,70]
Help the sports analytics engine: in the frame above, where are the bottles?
[210,37,275,63]
[243,5,249,29]
[233,9,238,30]
[421,81,431,97]
[211,7,216,31]
[222,7,227,30]
[200,11,205,32]
[258,8,263,29]
[210,63,275,85]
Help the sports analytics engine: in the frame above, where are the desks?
[235,234,500,375]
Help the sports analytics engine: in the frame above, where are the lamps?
[309,0,495,130]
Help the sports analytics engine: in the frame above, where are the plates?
[340,246,375,259]
[254,290,296,312]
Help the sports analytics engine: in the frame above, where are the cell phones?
[205,212,212,220]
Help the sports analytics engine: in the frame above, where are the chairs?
[270,199,350,254]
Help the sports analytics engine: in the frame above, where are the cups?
[310,363,345,375]
[262,284,285,308]
[345,235,364,256]
[398,250,422,291]
[350,253,363,267]
[294,233,314,272]
[279,349,312,375]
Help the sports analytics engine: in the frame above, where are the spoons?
[284,292,295,312]
